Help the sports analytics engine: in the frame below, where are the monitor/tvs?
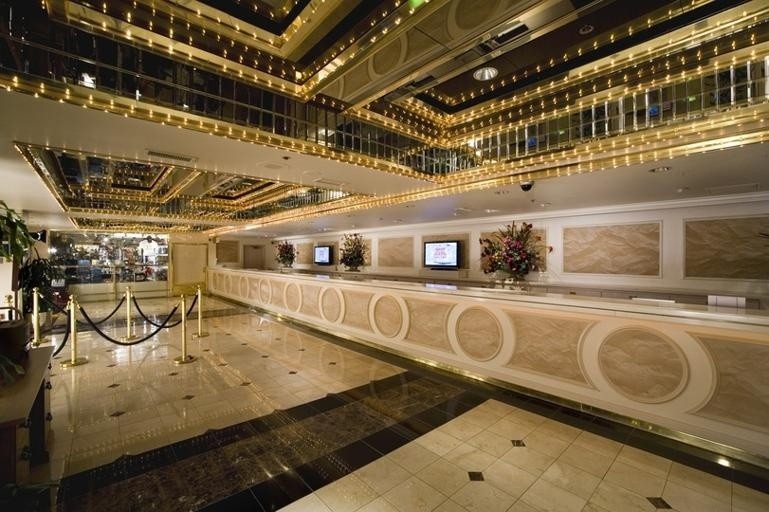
[424,241,459,267]
[314,246,331,265]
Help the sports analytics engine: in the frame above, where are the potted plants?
[340,235,365,271]
[18,254,58,333]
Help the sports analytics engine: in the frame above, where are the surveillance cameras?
[519,182,533,191]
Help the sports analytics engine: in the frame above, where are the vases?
[282,266,293,274]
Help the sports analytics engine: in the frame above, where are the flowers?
[276,241,295,266]
[477,221,553,277]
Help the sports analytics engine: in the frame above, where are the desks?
[1,345,55,483]
